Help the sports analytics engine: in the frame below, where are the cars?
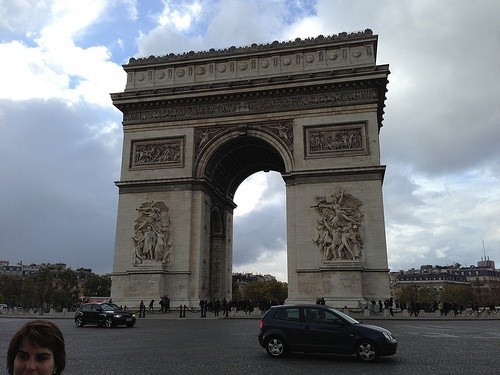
[259,303,398,362]
[74,302,136,330]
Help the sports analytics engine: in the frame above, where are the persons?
[138,300,143,312]
[199,297,281,315]
[148,300,154,313]
[195,128,220,153]
[471,302,495,313]
[371,297,394,316]
[159,296,170,314]
[131,206,171,264]
[267,122,293,146]
[395,300,465,317]
[310,189,363,260]
[316,297,325,305]
[7,320,66,375]
[109,299,112,303]
